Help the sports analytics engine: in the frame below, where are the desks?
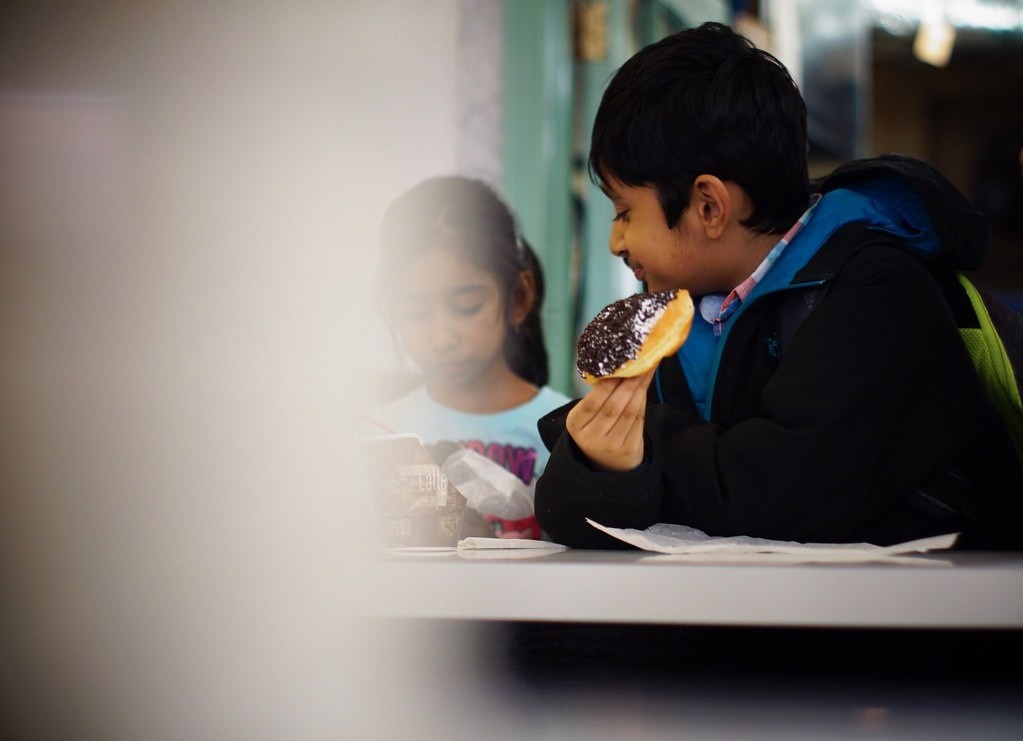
[346,543,1023,631]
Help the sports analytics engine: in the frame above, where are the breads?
[576,286,695,382]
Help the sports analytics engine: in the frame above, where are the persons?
[536,22,1023,741]
[376,174,574,542]
